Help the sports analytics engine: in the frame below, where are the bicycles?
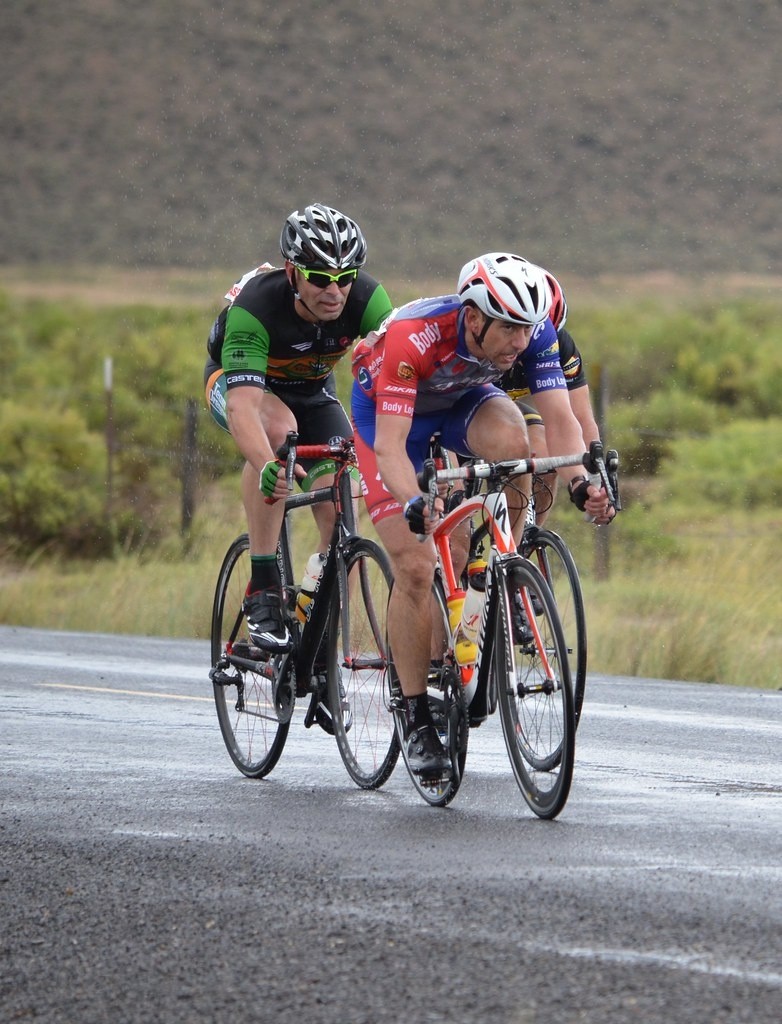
[210,431,448,787]
[426,451,633,770]
[383,433,619,822]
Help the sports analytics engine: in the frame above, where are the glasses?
[290,261,358,289]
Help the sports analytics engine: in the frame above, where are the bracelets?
[403,494,423,522]
[567,475,587,496]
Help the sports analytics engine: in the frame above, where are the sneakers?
[526,582,544,617]
[407,725,453,773]
[242,579,294,654]
[511,585,534,645]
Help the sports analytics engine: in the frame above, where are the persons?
[344,251,612,772]
[435,266,620,665]
[202,200,394,652]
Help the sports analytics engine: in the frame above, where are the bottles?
[294,554,327,623]
[459,566,494,643]
[446,584,481,665]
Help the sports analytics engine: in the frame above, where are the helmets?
[279,203,369,271]
[456,251,552,324]
[531,262,568,334]
